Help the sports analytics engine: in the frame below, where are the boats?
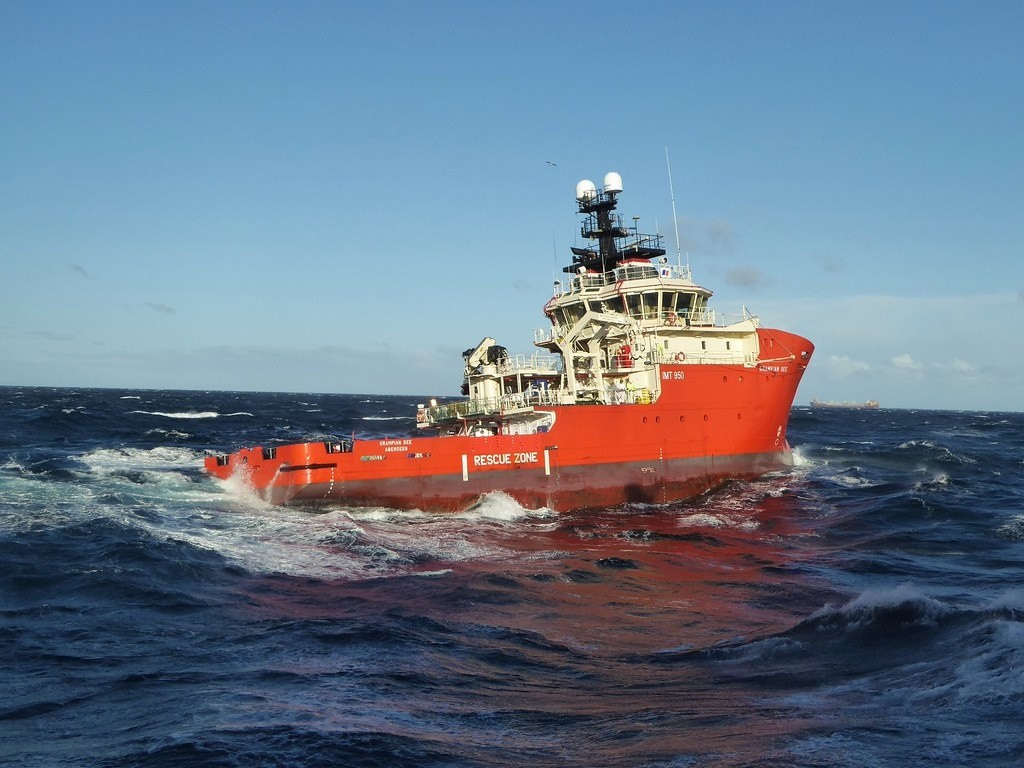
[203,172,816,516]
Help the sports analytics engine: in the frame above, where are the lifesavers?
[667,312,677,323]
[677,352,686,362]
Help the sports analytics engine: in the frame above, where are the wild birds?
[546,161,557,167]
